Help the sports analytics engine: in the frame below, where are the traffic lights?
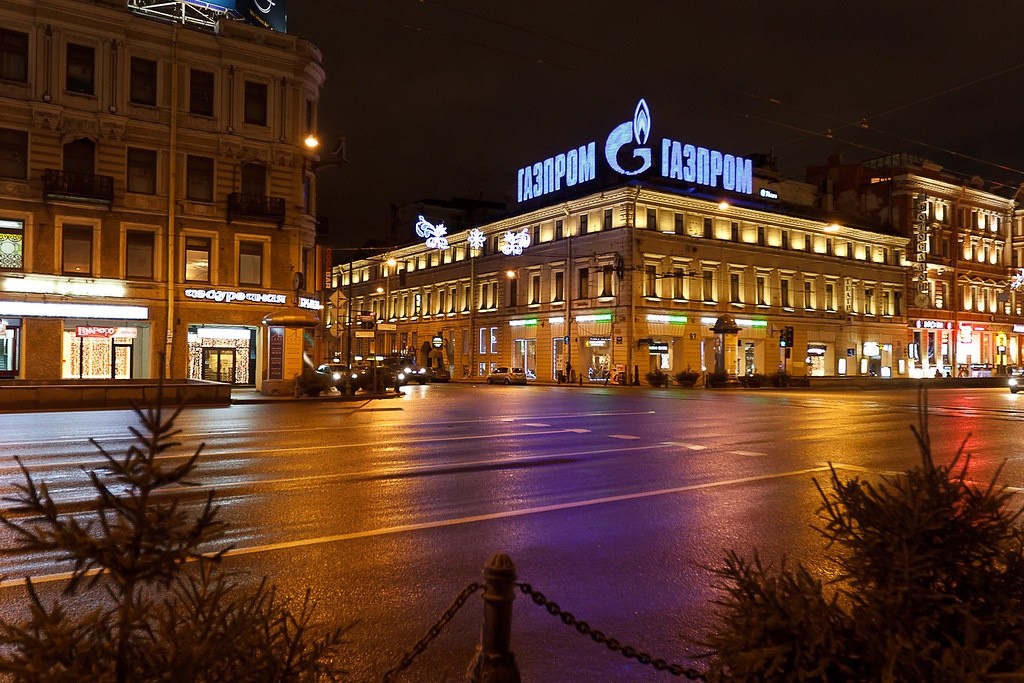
[780,329,787,347]
[787,327,793,347]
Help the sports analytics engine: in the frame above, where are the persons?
[565,362,571,382]
[963,368,969,377]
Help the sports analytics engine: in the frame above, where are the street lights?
[385,260,397,356]
[999,346,1005,376]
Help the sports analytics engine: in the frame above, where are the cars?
[349,366,399,389]
[1012,365,1024,377]
[425,367,451,383]
[315,364,350,385]
[486,366,527,385]
[378,358,429,385]
[1009,372,1024,394]
